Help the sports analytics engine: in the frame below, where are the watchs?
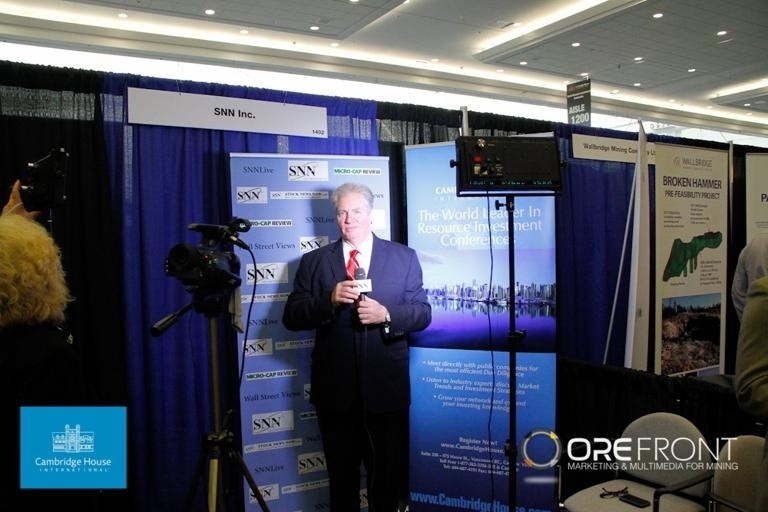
[382,312,391,331]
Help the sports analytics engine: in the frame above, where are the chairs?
[553,410,767,511]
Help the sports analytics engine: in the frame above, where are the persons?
[731,232,768,322]
[281,183,432,512]
[0,214,79,512]
[733,274,768,512]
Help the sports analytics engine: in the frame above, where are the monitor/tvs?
[457,135,564,192]
[0,391,138,510]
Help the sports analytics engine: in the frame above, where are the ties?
[345,250,363,306]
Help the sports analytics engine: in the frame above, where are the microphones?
[350,267,374,304]
